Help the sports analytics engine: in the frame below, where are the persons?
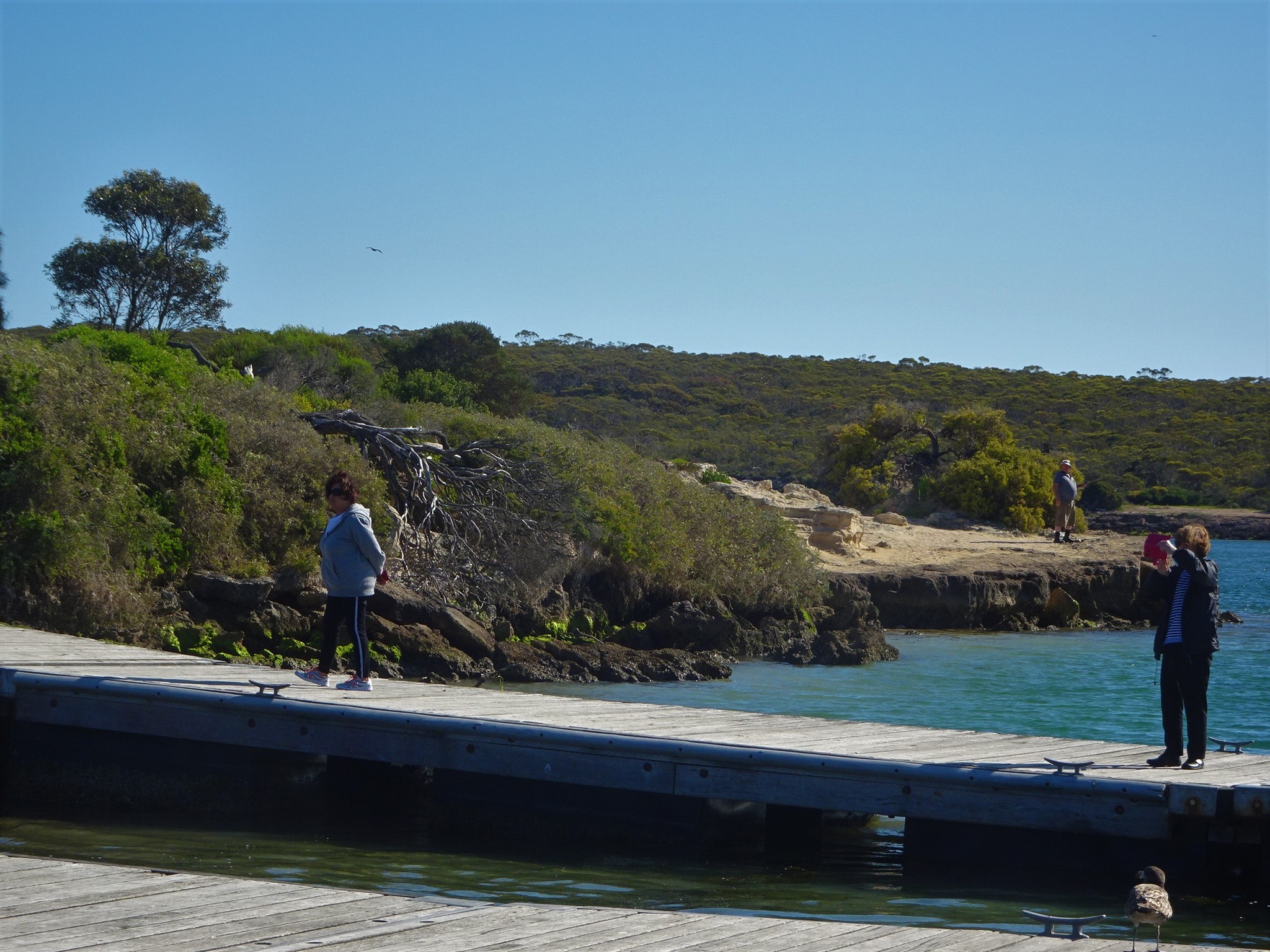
[1135,523,1221,770]
[295,466,386,691]
[1052,460,1088,543]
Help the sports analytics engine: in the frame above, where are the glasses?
[1174,541,1187,546]
[328,488,346,497]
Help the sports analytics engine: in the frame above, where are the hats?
[1059,460,1072,469]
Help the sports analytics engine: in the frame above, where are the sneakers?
[336,674,373,691]
[294,667,329,687]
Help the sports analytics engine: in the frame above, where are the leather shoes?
[1147,754,1182,767]
[1182,757,1204,769]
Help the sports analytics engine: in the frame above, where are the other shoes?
[1063,537,1075,542]
[1054,539,1065,543]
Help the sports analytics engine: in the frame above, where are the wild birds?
[1123,865,1173,952]
[366,247,382,253]
